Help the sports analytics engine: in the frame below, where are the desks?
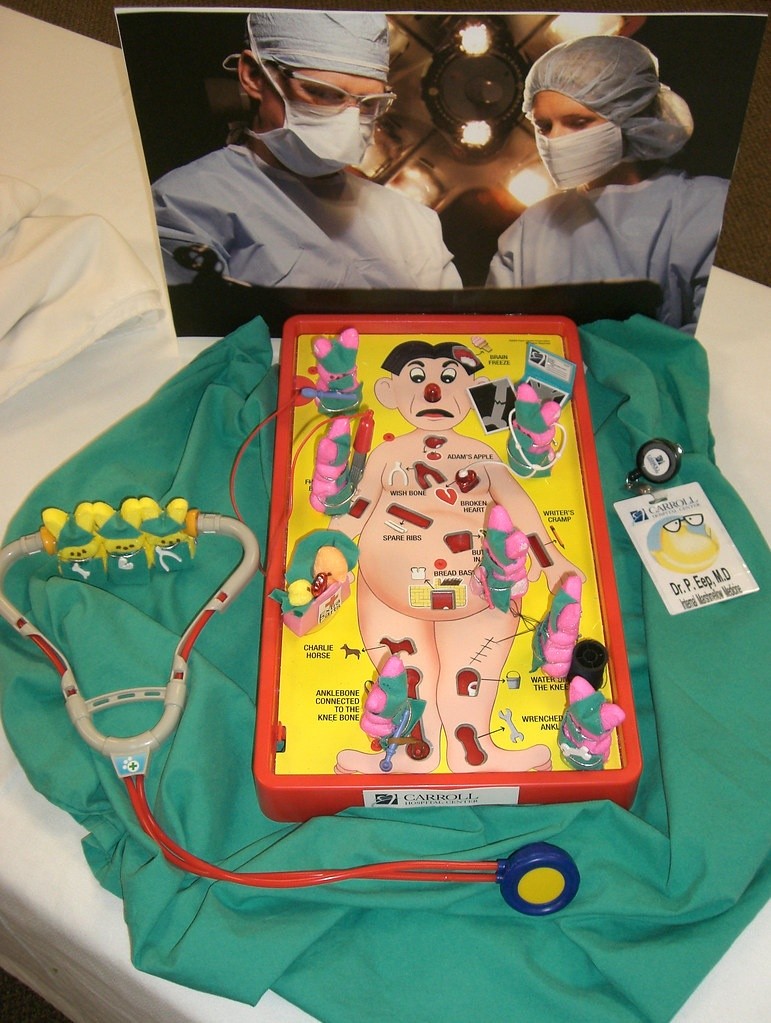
[0,0,769,1022]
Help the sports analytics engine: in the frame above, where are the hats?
[532,121,624,192]
[244,12,389,82]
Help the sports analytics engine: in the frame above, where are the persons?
[480,32,731,339]
[142,7,461,289]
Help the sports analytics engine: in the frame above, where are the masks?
[247,66,381,178]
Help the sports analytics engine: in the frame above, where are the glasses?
[272,61,397,119]
